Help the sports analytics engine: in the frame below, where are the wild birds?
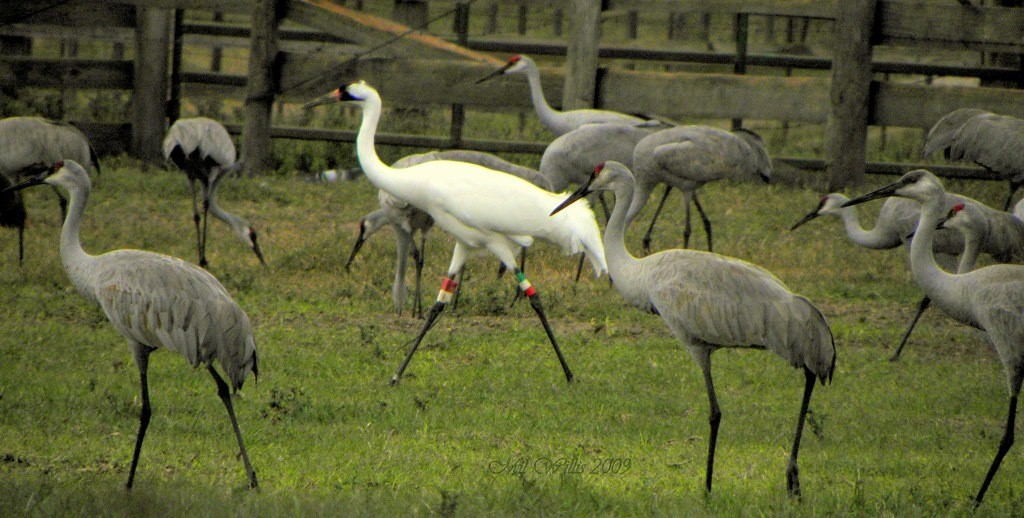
[791,105,1024,508]
[1,113,272,495]
[296,54,837,504]
